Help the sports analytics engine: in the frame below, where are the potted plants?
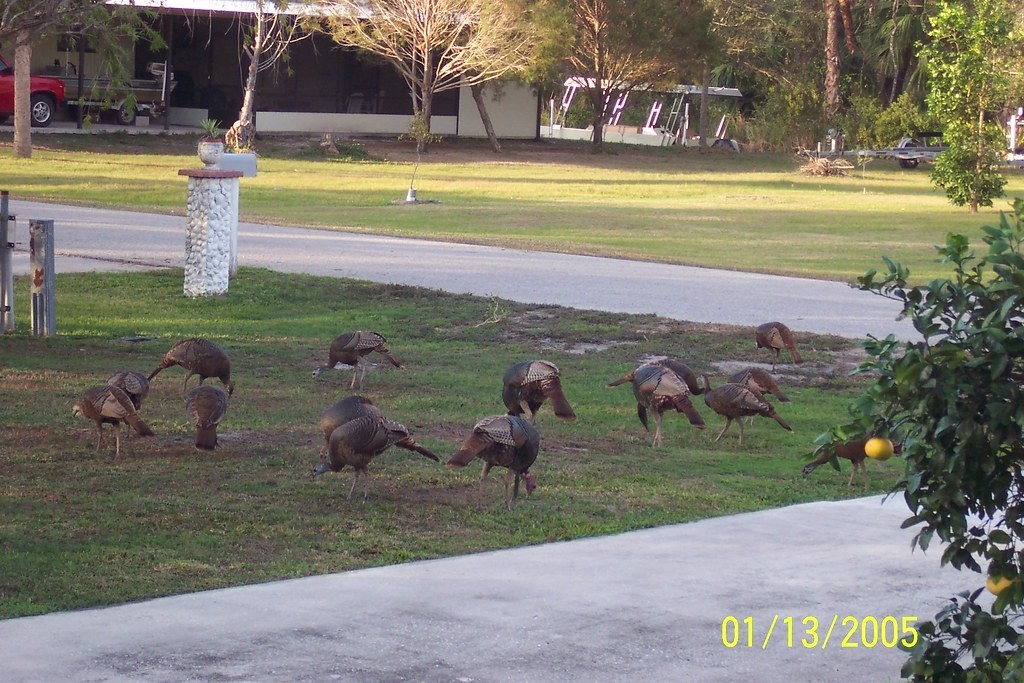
[198,118,223,142]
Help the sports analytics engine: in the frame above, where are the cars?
[0,54,67,127]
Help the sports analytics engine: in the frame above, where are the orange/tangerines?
[986,574,1017,596]
[865,437,893,460]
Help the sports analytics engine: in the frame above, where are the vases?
[197,142,223,170]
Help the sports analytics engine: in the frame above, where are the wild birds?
[503,359,577,423]
[319,396,381,463]
[633,366,706,449]
[312,331,407,391]
[697,371,793,445]
[106,372,149,438]
[311,414,440,503]
[72,385,154,460]
[606,359,710,395]
[726,365,790,426]
[446,415,540,511]
[754,321,804,372]
[802,430,904,490]
[148,338,234,397]
[185,384,226,451]
